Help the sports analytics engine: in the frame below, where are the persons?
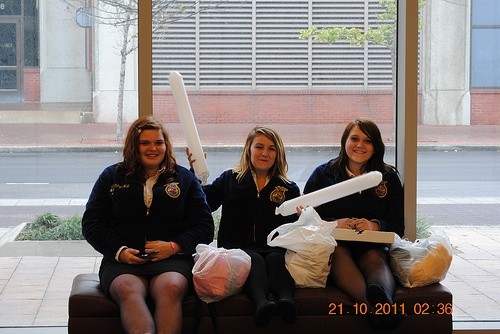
[82,115,214,334]
[304,118,405,330]
[186,125,309,327]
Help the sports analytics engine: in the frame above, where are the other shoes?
[278,298,297,324]
[254,302,276,327]
[366,284,394,314]
[369,312,407,334]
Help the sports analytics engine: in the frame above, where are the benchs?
[68,272,454,334]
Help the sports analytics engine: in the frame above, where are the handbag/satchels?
[388,228,452,289]
[267,205,337,290]
[191,240,252,306]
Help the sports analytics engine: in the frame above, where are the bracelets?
[170,241,176,255]
